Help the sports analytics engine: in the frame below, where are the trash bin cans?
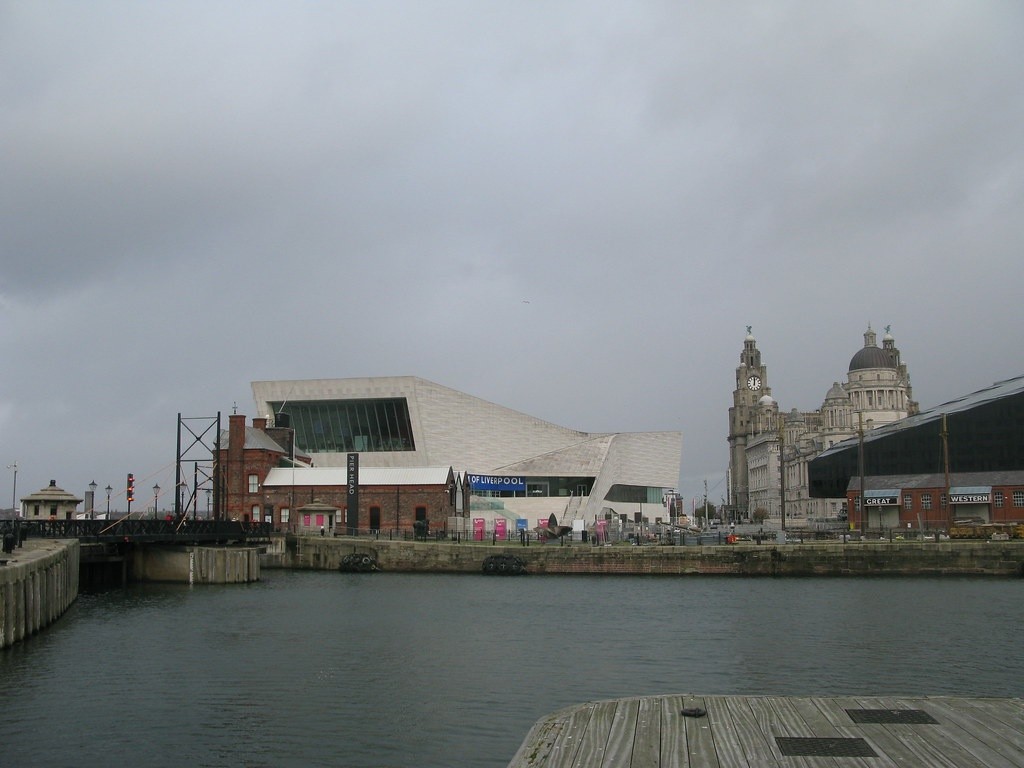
[582,530,587,541]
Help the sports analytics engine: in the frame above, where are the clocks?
[747,376,762,391]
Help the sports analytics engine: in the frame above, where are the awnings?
[864,489,902,506]
[949,486,992,504]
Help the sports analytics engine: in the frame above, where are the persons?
[166,512,171,520]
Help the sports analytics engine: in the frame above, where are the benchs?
[748,533,776,542]
[788,530,847,544]
[890,530,943,542]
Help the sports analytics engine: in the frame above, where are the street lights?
[7,460,19,533]
[153,483,160,519]
[205,488,212,520]
[178,482,188,520]
[105,484,113,520]
[88,480,97,521]
